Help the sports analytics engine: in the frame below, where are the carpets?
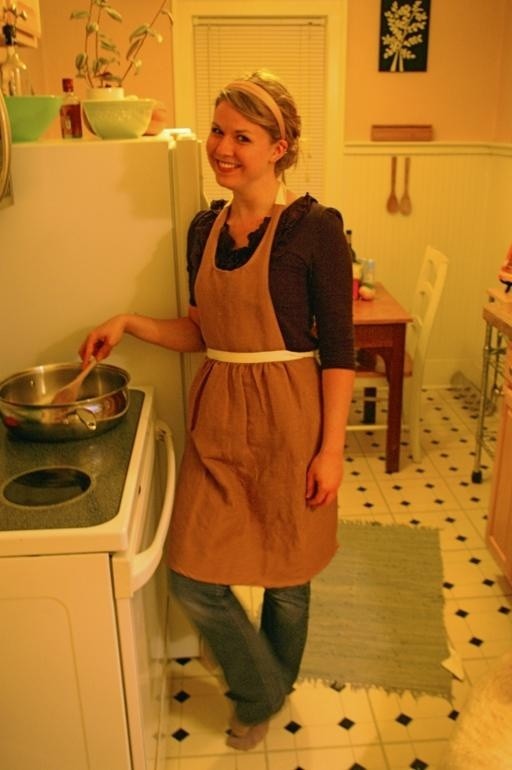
[303,518,455,704]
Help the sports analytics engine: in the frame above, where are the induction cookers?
[0,385,149,533]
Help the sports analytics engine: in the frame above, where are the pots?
[1,361,133,444]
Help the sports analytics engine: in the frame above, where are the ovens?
[109,415,178,770]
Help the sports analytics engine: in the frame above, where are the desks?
[309,280,413,474]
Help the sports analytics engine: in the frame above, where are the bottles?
[1,22,32,96]
[58,78,83,139]
[346,229,378,302]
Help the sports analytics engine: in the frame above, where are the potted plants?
[68,0,181,136]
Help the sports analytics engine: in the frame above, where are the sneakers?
[227,715,272,751]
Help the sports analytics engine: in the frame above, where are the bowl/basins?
[80,96,158,139]
[2,93,66,143]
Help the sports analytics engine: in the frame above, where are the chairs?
[345,243,451,466]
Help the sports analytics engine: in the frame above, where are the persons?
[79,76,356,749]
